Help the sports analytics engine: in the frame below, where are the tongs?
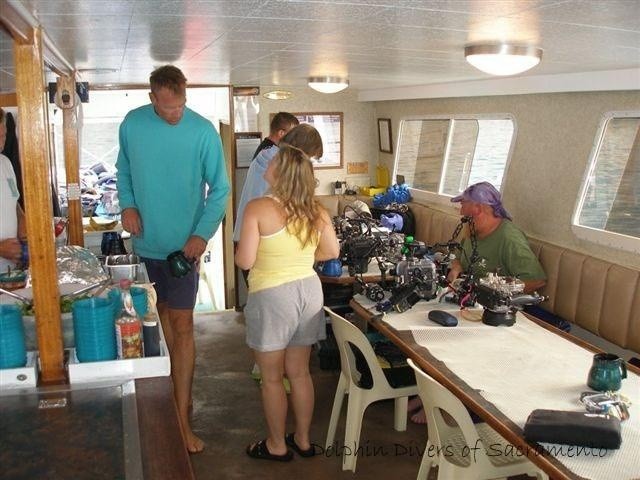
[60,278,114,302]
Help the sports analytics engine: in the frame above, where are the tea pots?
[584,351,628,393]
[166,251,195,278]
[322,259,343,279]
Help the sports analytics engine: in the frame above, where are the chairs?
[405,356,547,479]
[320,302,420,475]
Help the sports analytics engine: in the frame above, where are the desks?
[313,259,403,286]
[347,284,639,480]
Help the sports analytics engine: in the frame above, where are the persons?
[233,145,343,463]
[115,63,232,456]
[251,111,299,160]
[408,182,549,425]
[234,123,323,395]
[0,110,27,276]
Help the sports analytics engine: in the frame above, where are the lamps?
[307,76,350,97]
[464,42,543,80]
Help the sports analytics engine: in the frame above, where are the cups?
[1,304,27,368]
[71,297,116,361]
[101,232,126,255]
[109,285,148,312]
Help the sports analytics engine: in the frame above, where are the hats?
[451,182,501,206]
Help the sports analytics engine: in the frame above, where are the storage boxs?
[358,164,388,196]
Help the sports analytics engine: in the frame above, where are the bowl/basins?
[481,309,518,327]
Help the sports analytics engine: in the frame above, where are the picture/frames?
[376,118,394,154]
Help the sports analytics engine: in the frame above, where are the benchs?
[314,194,379,221]
[392,196,638,365]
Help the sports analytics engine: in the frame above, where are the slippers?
[245,435,295,463]
[283,431,316,458]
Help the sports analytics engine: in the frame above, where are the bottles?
[143,313,162,356]
[396,257,437,297]
[115,278,143,357]
